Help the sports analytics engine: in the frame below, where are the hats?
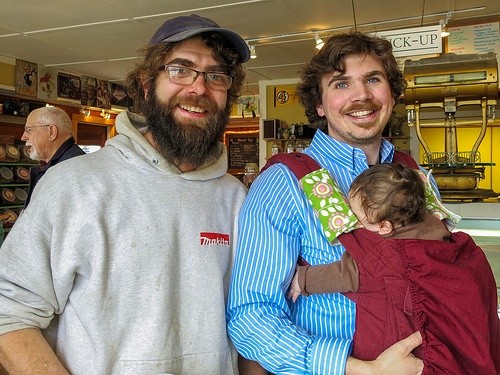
[148,14,250,63]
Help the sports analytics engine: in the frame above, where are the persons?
[21,107,86,209]
[288,163,500,375]
[226,31,445,375]
[0,14,272,375]
[88,77,110,107]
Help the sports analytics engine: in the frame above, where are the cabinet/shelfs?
[0,88,46,250]
[263,138,312,160]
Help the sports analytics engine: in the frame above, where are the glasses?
[159,64,233,90]
[24,124,50,133]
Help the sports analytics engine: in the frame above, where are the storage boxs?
[302,124,316,139]
[264,118,280,139]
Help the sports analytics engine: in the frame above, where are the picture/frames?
[128,86,135,108]
[97,79,111,110]
[111,82,128,108]
[57,72,81,101]
[38,64,57,103]
[80,75,98,107]
[14,59,39,98]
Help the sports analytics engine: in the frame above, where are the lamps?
[80,107,91,118]
[246,41,257,59]
[439,13,454,38]
[313,32,325,51]
[99,109,111,120]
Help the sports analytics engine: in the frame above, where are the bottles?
[243,163,259,188]
[286,140,307,153]
[271,141,283,157]
[291,122,304,134]
